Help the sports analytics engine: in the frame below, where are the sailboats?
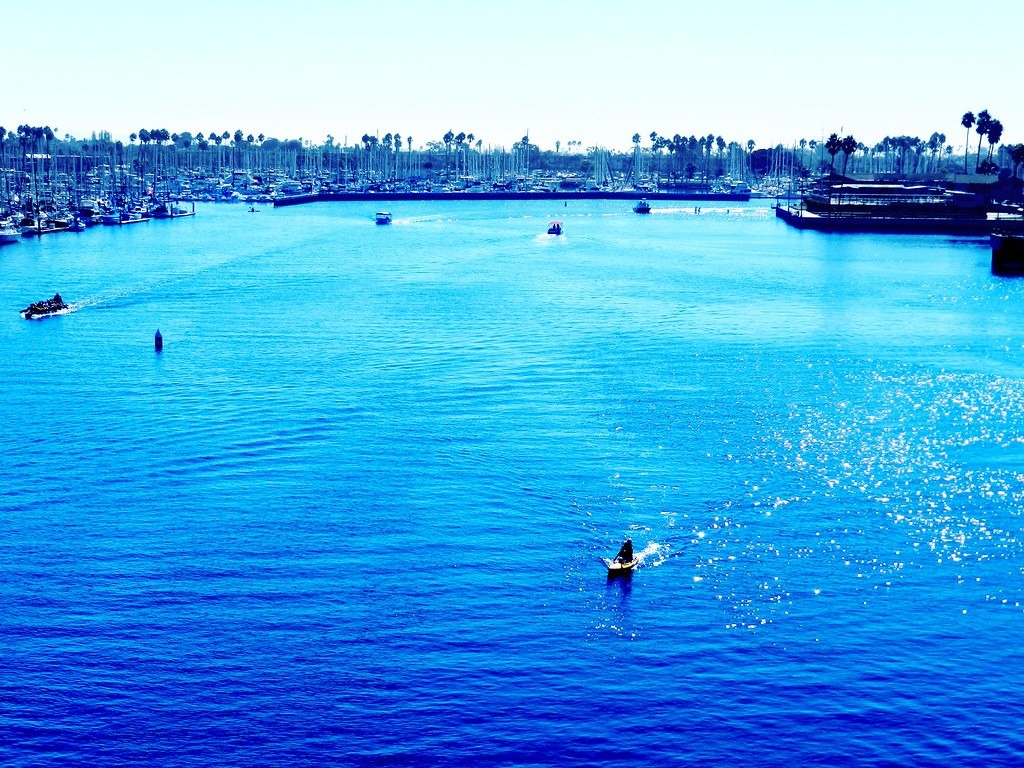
[0,136,831,245]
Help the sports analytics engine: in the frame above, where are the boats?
[548,220,563,235]
[632,198,651,214]
[19,303,70,320]
[608,556,638,573]
[248,209,260,213]
[375,210,392,223]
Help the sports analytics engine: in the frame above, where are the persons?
[553,224,559,229]
[620,540,633,563]
[30,293,63,312]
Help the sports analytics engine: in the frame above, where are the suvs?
[1006,205,1023,215]
